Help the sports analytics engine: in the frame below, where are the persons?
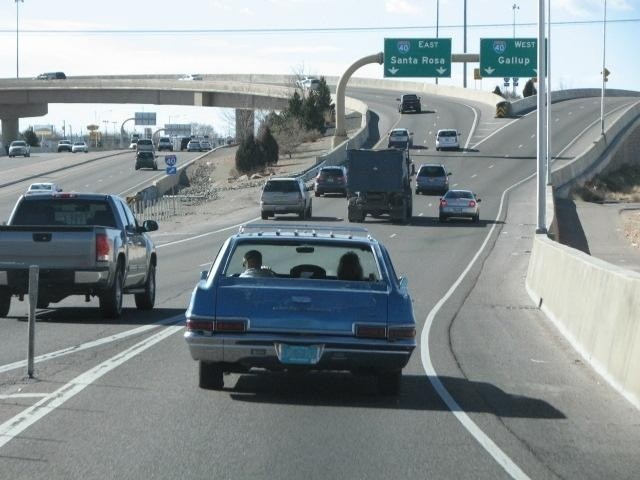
[238,249,273,279]
[336,251,360,279]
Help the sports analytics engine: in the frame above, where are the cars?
[395,93,423,116]
[301,79,320,90]
[24,181,62,196]
[8,140,32,158]
[57,139,72,152]
[245,125,492,224]
[129,133,213,152]
[177,73,202,80]
[295,75,314,88]
[184,225,418,396]
[134,150,159,172]
[72,141,90,153]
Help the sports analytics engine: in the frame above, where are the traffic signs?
[383,37,453,79]
[479,37,548,79]
[165,155,176,165]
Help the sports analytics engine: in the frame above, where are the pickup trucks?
[0,191,163,322]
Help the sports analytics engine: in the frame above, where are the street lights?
[14,0,26,78]
[510,2,521,38]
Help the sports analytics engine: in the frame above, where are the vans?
[33,72,67,79]
